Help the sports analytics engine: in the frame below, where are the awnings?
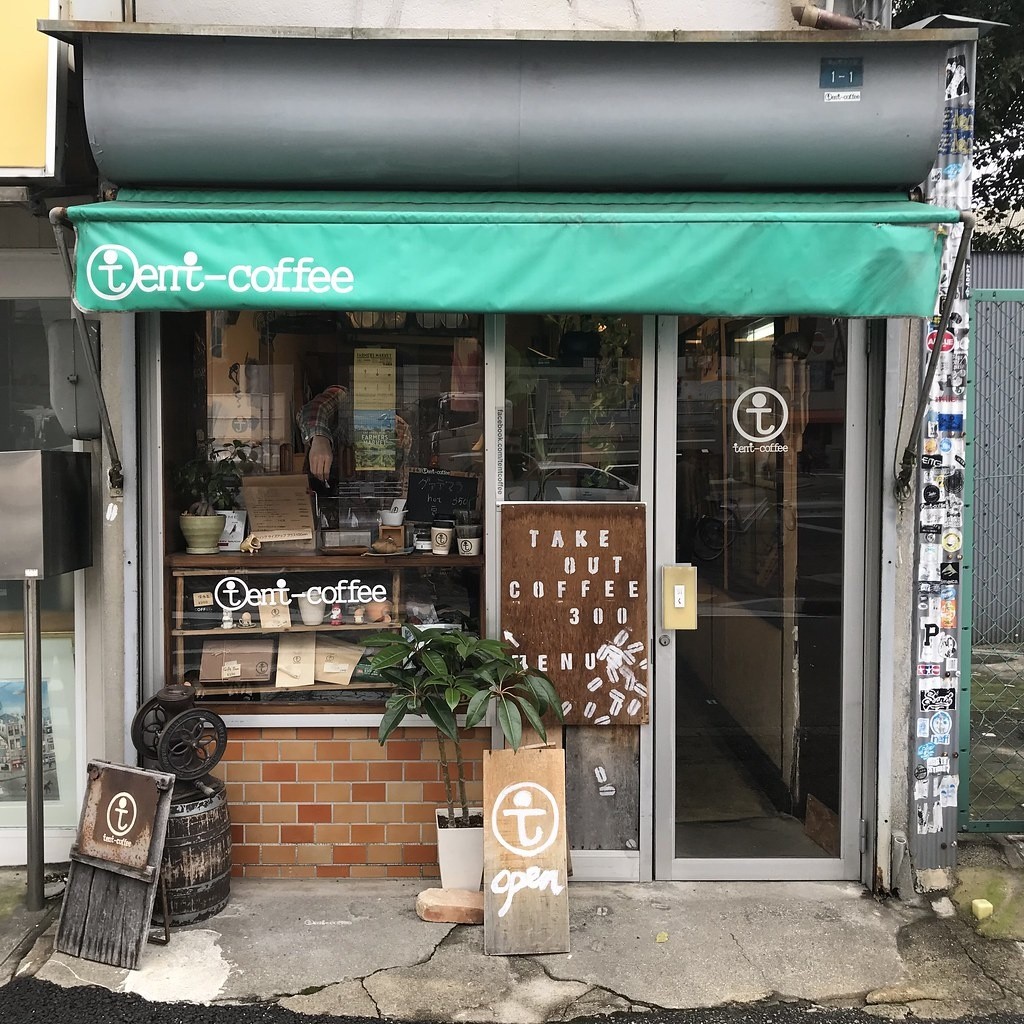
[46,187,971,317]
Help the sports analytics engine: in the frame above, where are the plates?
[416,313,463,329]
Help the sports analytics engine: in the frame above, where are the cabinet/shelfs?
[164,553,486,699]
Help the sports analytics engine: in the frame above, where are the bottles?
[416,534,432,552]
[413,523,431,549]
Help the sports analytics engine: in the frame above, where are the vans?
[536,462,640,501]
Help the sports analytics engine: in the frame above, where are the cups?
[431,514,455,555]
[298,591,333,626]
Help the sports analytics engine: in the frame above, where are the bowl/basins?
[350,312,407,328]
[376,510,409,527]
[454,509,482,556]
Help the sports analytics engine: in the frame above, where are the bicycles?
[689,473,799,562]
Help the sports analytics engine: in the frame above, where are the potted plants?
[354,618,564,889]
[166,437,261,553]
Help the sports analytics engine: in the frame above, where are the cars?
[605,464,640,486]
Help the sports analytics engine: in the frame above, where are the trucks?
[431,393,724,482]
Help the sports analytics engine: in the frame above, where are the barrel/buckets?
[148,775,231,928]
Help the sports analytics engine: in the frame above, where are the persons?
[292,362,413,518]
